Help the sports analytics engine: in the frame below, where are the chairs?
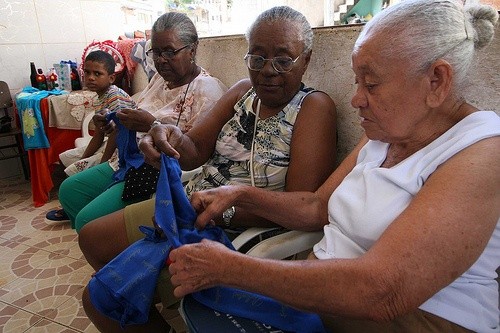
[0,81,30,183]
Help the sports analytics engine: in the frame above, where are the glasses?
[243,43,311,73]
[146,45,188,59]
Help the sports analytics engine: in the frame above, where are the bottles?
[37,69,48,90]
[47,68,56,89]
[29,62,39,89]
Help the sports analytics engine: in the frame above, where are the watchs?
[221,203,235,228]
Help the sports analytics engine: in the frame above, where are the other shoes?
[46,209,69,221]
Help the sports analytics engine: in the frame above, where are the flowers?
[36,74,46,90]
[50,73,59,89]
[71,73,77,80]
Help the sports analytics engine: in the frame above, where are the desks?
[13,85,100,208]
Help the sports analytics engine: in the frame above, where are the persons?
[45,49,142,222]
[77,6,338,333]
[58,13,232,248]
[172,0,500,332]
